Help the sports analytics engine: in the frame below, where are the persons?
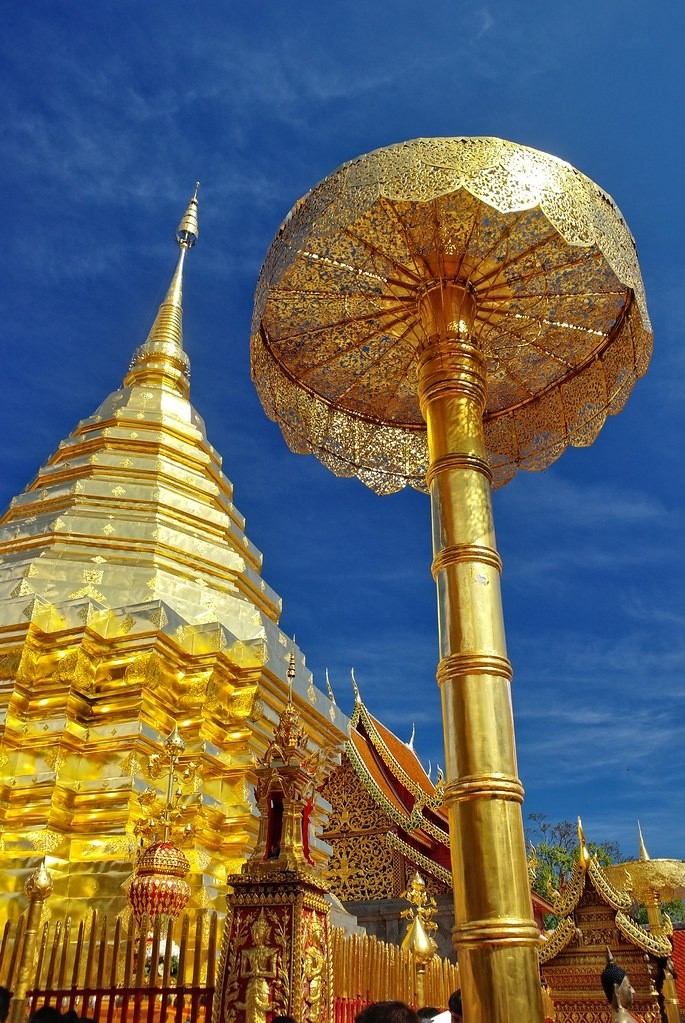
[270,1016,298,1023]
[448,989,464,1023]
[417,1006,439,1023]
[600,946,647,1023]
[239,907,279,1023]
[0,986,98,1023]
[354,1000,418,1023]
